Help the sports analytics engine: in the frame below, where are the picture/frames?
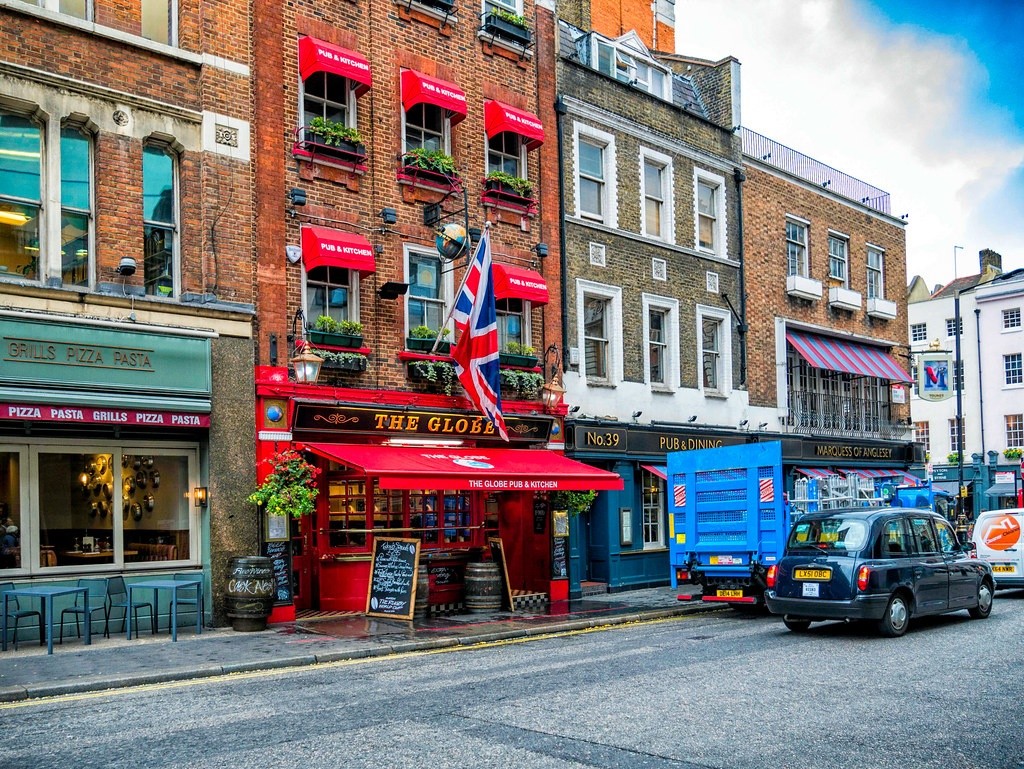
[348,487,365,512]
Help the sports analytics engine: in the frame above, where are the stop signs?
[1020,461,1024,480]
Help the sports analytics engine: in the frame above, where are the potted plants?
[486,7,531,44]
[306,316,545,400]
[306,116,532,204]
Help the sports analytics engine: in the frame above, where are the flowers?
[1004,447,1024,459]
[246,449,322,519]
[551,489,599,516]
[949,452,966,463]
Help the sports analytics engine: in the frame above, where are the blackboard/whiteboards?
[550,501,570,580]
[488,537,515,612]
[366,536,421,620]
[257,501,294,607]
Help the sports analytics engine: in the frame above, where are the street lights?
[954,268,1024,548]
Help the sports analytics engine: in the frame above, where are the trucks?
[667,440,958,618]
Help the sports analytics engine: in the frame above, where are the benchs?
[127,543,179,560]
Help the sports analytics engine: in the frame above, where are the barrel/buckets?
[463,563,502,613]
[223,556,276,631]
[413,564,429,618]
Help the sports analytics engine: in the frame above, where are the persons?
[419,499,433,511]
[0,502,20,569]
[348,498,355,512]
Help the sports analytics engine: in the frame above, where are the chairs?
[0,572,205,651]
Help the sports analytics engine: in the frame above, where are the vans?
[968,507,1024,590]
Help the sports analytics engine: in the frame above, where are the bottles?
[105,537,111,549]
[73,538,79,551]
[94,538,100,552]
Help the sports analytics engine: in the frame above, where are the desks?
[63,551,138,563]
[1,585,89,656]
[127,579,201,642]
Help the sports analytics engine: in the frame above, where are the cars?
[764,507,997,638]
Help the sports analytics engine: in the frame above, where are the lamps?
[530,243,548,258]
[468,227,481,243]
[287,306,326,386]
[375,282,409,300]
[569,406,768,427]
[287,189,308,206]
[540,341,568,412]
[377,208,396,223]
[193,487,208,508]
[115,257,137,275]
[387,437,463,445]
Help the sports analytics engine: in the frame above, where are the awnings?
[484,99,546,153]
[300,227,376,279]
[642,465,787,501]
[401,70,468,128]
[786,328,917,386]
[837,470,943,492]
[983,480,1023,497]
[298,36,372,100]
[291,441,624,491]
[796,469,845,480]
[491,263,550,310]
[931,480,974,499]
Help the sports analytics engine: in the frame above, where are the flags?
[450,228,508,444]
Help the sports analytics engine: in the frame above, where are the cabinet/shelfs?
[144,228,172,285]
